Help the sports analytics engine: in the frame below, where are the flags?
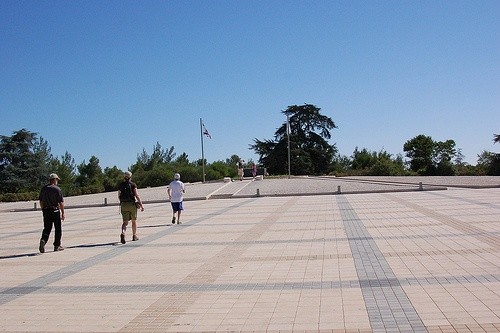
[286,118,292,136]
[201,120,211,138]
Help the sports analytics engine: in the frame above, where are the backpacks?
[121,182,133,202]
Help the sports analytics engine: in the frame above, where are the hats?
[50,174,60,180]
[124,170,132,177]
[175,174,180,180]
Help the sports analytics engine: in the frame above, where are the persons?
[236,160,244,181]
[167,173,185,224]
[117,171,144,244]
[38,173,65,253]
[251,163,256,179]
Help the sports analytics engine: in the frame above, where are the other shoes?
[39,240,45,253]
[54,246,64,250]
[121,234,125,244]
[133,236,139,240]
[172,217,176,223]
[178,222,182,225]
